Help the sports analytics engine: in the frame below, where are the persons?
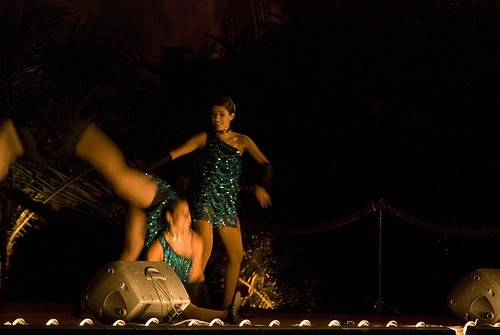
[145,198,205,313]
[66,116,180,265]
[142,96,272,312]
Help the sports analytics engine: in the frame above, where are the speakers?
[447,267,499,326]
[81,261,191,326]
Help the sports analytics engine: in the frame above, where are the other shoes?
[236,308,252,320]
[225,293,242,324]
